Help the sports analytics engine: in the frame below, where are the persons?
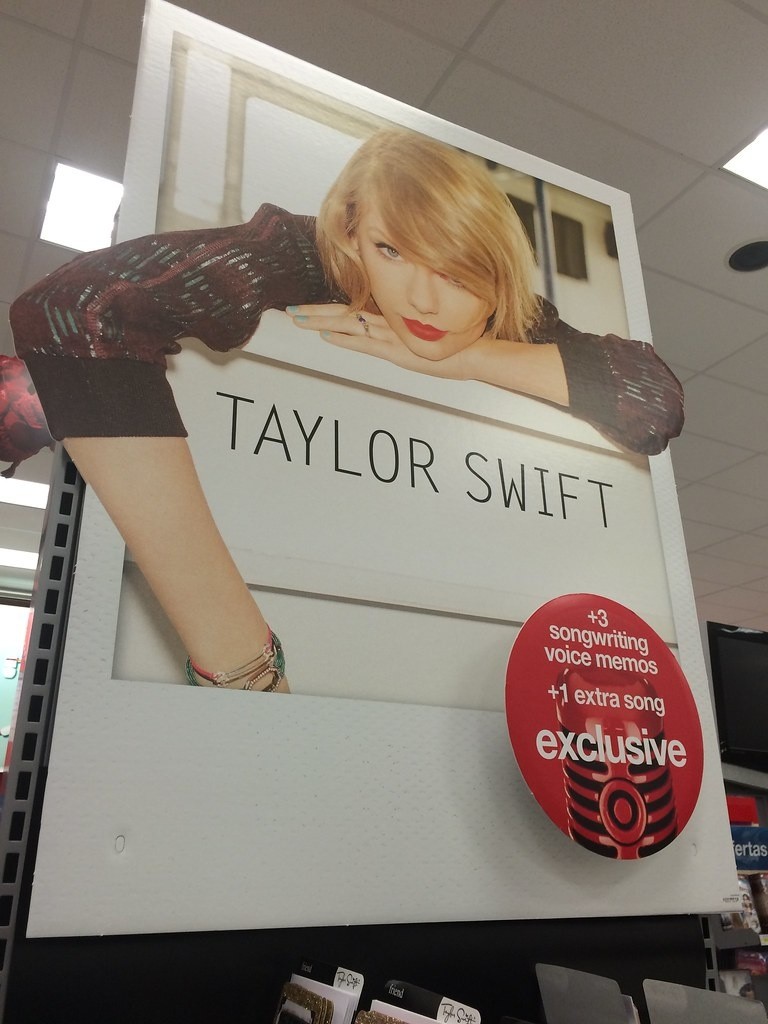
[9,127,686,695]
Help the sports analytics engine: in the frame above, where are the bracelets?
[183,630,286,694]
[185,621,274,688]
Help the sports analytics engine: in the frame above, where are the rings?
[355,312,374,337]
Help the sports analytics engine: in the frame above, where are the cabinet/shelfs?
[699,762,768,1005]
